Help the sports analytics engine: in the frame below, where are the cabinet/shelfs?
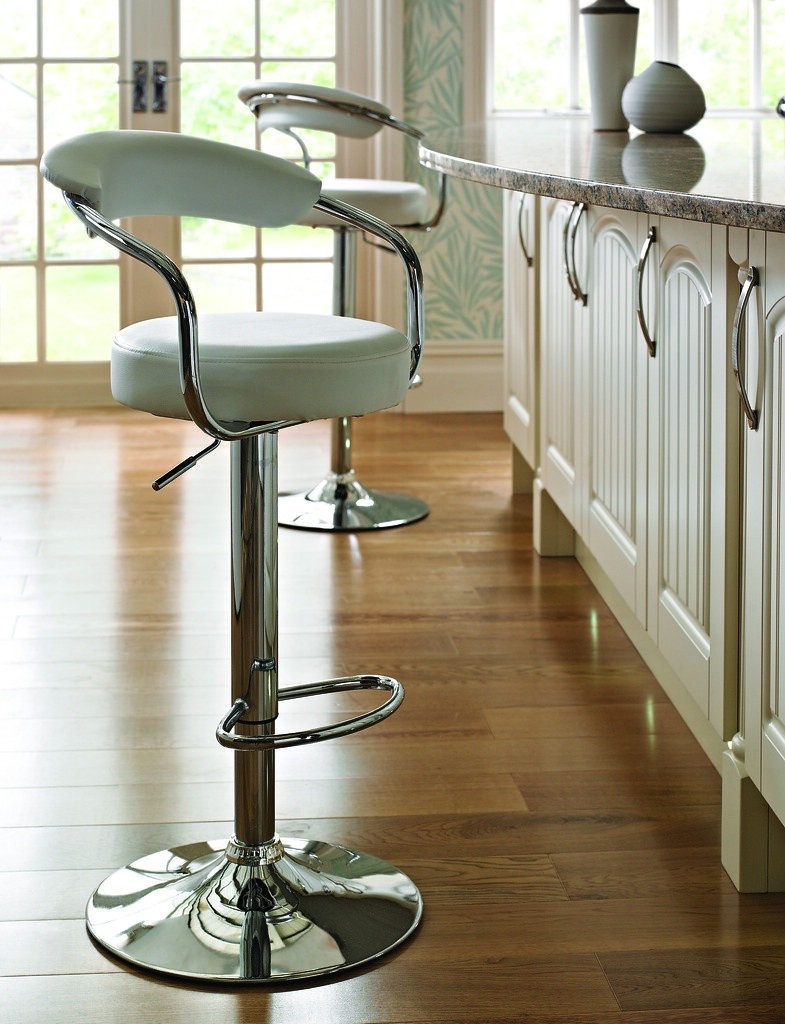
[633,206,742,744]
[730,227,785,829]
[537,194,649,632]
[499,187,538,469]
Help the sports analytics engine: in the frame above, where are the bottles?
[620,133,706,192]
[620,60,706,133]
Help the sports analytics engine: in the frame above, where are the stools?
[235,78,448,530]
[34,125,431,994]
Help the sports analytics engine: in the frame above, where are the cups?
[580,0,640,132]
